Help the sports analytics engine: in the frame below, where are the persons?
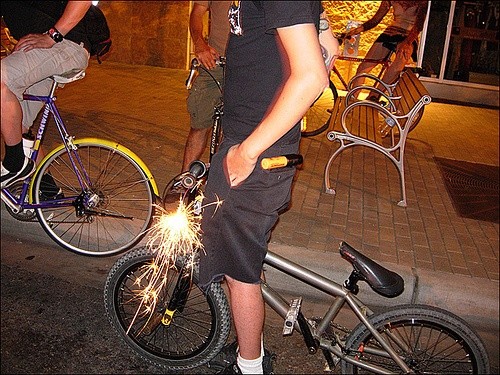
[170,1,238,194]
[1,0,101,206]
[341,1,429,106]
[198,1,339,374]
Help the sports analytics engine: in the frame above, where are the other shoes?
[204,339,279,374]
[165,186,189,204]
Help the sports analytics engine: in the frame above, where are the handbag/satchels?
[73,4,113,65]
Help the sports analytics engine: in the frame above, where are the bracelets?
[48,27,63,43]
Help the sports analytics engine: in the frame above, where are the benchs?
[324,67,432,207]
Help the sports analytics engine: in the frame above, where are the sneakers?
[14,187,65,205]
[1,155,38,190]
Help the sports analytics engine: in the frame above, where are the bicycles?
[300,34,432,138]
[184,56,228,159]
[0,50,162,255]
[103,158,492,375]
